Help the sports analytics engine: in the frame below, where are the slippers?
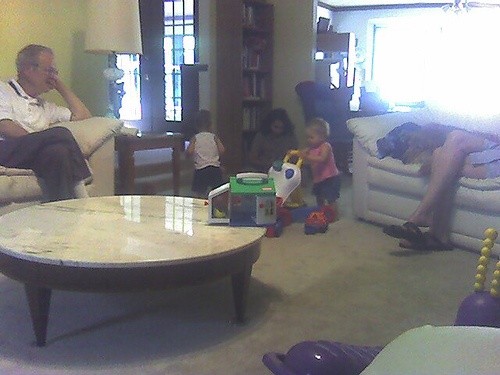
[384,221,428,247]
[399,233,451,251]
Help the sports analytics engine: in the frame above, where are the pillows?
[53,116,123,158]
[346,108,434,159]
[439,109,499,136]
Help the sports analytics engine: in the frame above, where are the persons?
[186,110,225,199]
[383,130,500,251]
[1,44,92,203]
[248,108,298,169]
[286,117,343,210]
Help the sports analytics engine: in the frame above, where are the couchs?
[346,105,499,258]
[0,135,122,216]
[296,81,385,172]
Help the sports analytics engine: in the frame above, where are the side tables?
[115,132,184,195]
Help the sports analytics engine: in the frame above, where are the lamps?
[83,0,143,136]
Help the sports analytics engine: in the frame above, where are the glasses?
[32,62,58,77]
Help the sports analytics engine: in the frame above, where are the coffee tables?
[0,197,266,347]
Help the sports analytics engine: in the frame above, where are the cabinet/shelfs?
[212,1,274,173]
[316,29,354,87]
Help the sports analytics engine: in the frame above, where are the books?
[243,3,266,130]
